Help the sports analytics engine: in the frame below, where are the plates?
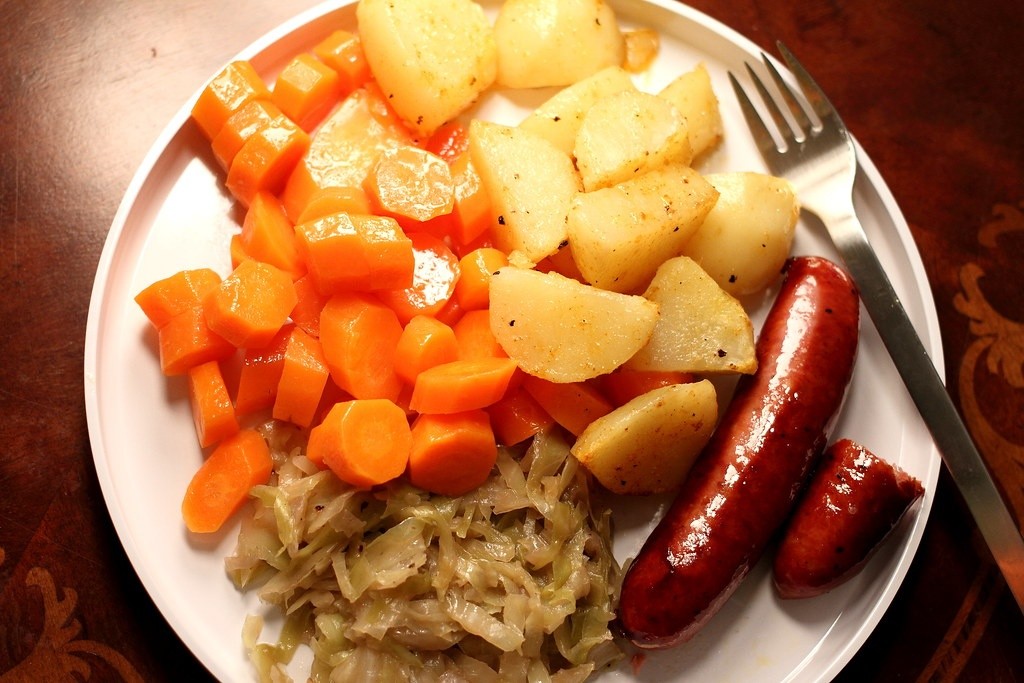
[85,0,945,681]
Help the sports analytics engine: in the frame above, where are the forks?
[726,40,1024,618]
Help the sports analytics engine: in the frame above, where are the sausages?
[618,256,926,671]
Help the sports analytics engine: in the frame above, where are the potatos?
[356,0,801,495]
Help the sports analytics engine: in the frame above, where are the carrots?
[134,27,693,536]
